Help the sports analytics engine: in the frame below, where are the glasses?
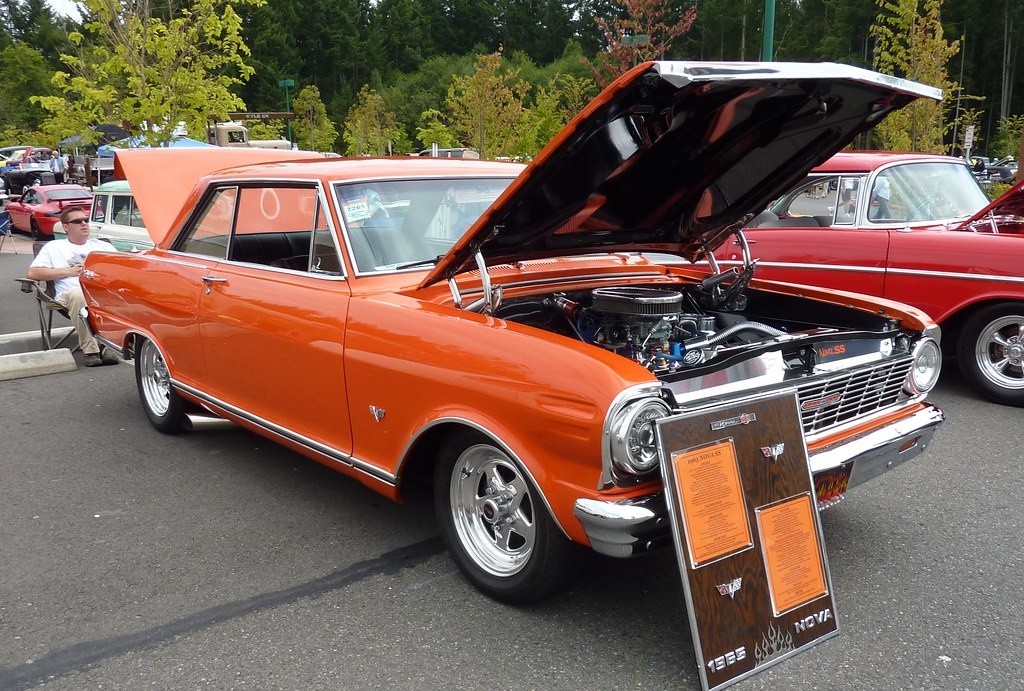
[64,216,89,224]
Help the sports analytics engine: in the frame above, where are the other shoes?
[80,354,103,367]
[99,347,119,364]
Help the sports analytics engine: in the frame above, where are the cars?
[76,62,948,613]
[0,121,391,273]
[647,150,1024,413]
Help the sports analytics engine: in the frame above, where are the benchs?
[743,210,779,229]
[187,225,425,278]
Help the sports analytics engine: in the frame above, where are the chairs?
[757,216,821,230]
[0,210,19,256]
[13,238,115,355]
[813,215,854,227]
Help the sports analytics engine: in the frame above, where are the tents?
[97,126,220,187]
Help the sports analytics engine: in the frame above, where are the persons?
[870,174,894,221]
[48,151,69,185]
[975,159,985,172]
[27,204,123,366]
[827,177,855,220]
